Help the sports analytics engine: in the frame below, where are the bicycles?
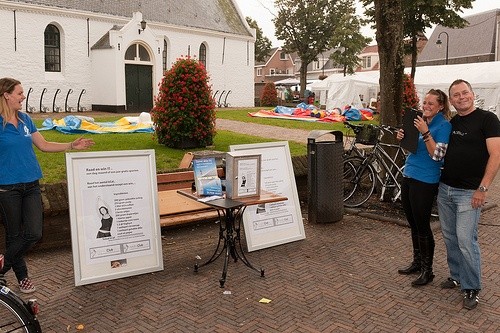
[340,120,443,220]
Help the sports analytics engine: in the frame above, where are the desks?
[158,185,288,288]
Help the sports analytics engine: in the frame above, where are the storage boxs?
[225,150,262,199]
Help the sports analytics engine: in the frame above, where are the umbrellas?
[274,79,313,91]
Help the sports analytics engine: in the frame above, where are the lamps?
[138,19,147,34]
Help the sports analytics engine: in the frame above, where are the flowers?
[148,58,217,146]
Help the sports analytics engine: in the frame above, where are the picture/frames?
[229,141,307,252]
[66,150,164,287]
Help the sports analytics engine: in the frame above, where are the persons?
[0,77,96,293]
[397,89,452,287]
[449,79,500,310]
[241,176,246,187]
[95,195,113,238]
[377,92,380,111]
[284,85,315,104]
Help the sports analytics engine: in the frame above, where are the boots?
[399,249,421,273]
[412,256,435,285]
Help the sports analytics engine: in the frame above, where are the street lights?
[435,31,449,64]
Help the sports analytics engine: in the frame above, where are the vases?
[165,135,204,148]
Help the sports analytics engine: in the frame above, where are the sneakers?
[19,278,35,293]
[441,277,460,288]
[463,289,479,310]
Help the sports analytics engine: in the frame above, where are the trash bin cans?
[306,129,345,225]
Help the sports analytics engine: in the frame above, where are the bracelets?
[422,130,431,143]
[68,142,73,150]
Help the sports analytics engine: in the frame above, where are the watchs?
[479,185,487,191]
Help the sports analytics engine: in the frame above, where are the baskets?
[357,124,379,145]
